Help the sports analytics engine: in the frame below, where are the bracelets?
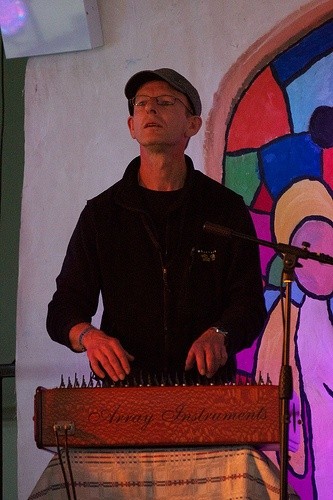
[79,325,96,353]
[210,326,228,337]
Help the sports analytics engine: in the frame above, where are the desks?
[27,446,301,500]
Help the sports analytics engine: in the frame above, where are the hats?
[125,68,202,116]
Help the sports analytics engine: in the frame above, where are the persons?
[46,67,268,387]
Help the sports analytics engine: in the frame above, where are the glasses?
[130,95,195,116]
